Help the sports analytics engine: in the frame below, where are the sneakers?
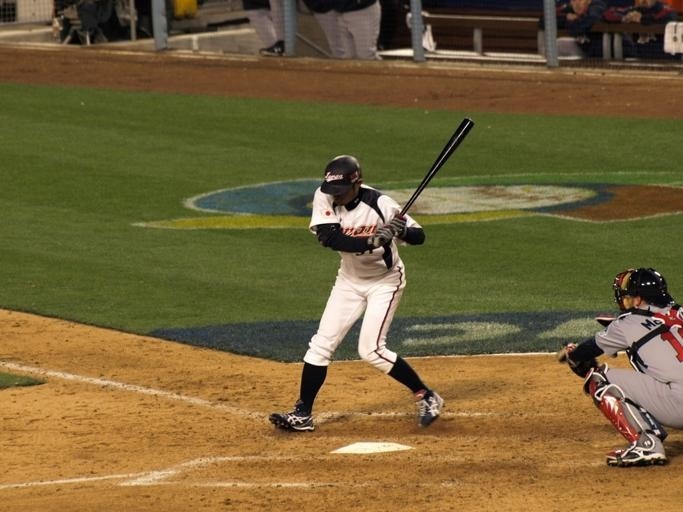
[414,389,444,426]
[268,403,315,433]
[606,430,666,468]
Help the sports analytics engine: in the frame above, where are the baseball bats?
[375,119,474,246]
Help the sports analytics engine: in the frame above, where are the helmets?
[321,154,363,196]
[612,265,673,314]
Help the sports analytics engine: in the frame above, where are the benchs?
[408,1,682,62]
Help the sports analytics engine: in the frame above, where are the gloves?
[390,214,407,241]
[366,226,394,250]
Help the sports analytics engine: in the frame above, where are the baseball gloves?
[559,342,598,377]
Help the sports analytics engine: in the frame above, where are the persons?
[556,267,682,466]
[244,0,286,56]
[270,155,445,432]
[539,0,683,61]
[305,0,382,62]
[52,0,153,45]
